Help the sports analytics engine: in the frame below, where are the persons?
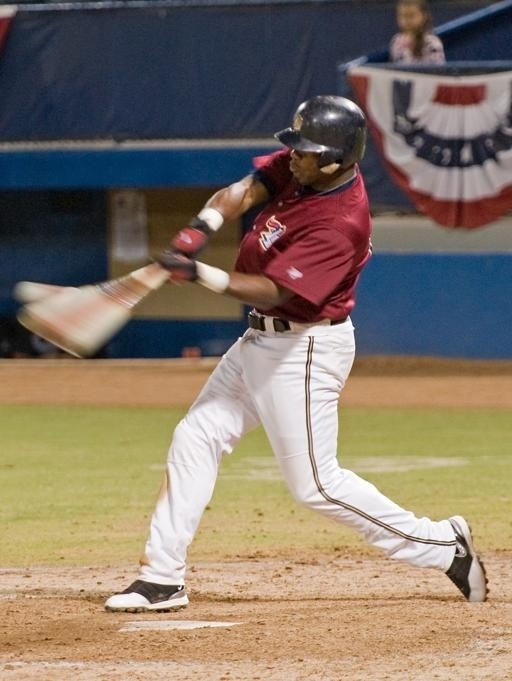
[103,92,491,612]
[389,0,446,64]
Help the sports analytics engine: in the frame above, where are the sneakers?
[444,516,490,605]
[103,574,190,613]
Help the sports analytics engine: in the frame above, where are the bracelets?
[195,259,230,299]
[188,206,224,237]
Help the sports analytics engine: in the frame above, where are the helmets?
[272,94,370,179]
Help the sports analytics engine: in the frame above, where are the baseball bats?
[17,262,171,358]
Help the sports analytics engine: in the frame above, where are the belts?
[244,307,350,332]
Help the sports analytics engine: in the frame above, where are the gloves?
[171,215,213,256]
[147,250,198,286]
